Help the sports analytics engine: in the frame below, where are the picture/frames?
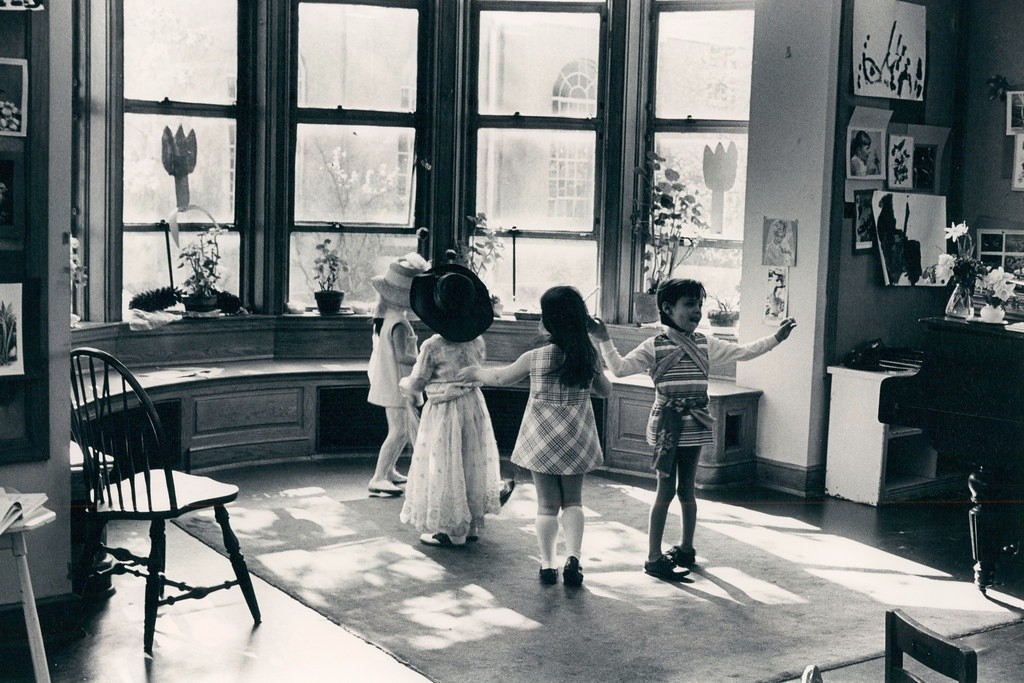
[1005,91,1024,194]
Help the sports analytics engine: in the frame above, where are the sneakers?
[391,474,408,484]
[368,478,403,497]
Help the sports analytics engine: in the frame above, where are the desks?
[0,486,57,683]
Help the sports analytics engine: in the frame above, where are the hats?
[409,264,495,343]
[371,251,428,308]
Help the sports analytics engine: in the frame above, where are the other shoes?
[563,556,585,588]
[539,564,560,585]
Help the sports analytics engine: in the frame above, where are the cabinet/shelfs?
[823,361,968,507]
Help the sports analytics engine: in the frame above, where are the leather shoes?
[665,545,696,567]
[644,555,690,579]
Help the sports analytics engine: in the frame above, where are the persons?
[367,257,429,495]
[457,287,612,587]
[589,279,797,578]
[764,222,791,266]
[851,130,873,176]
[398,264,500,547]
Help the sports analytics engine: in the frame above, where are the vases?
[979,301,1007,322]
[944,285,974,318]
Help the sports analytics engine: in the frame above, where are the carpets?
[170,458,1024,683]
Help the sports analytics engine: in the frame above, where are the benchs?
[71,323,763,491]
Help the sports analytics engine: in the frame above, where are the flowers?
[443,214,505,278]
[935,222,1018,309]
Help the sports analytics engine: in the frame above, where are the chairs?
[69,346,262,657]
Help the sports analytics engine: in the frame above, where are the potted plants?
[312,240,348,316]
[627,146,709,329]
[179,226,228,312]
[707,297,741,329]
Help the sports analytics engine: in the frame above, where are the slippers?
[421,532,467,548]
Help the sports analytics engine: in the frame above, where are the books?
[0,493,48,535]
